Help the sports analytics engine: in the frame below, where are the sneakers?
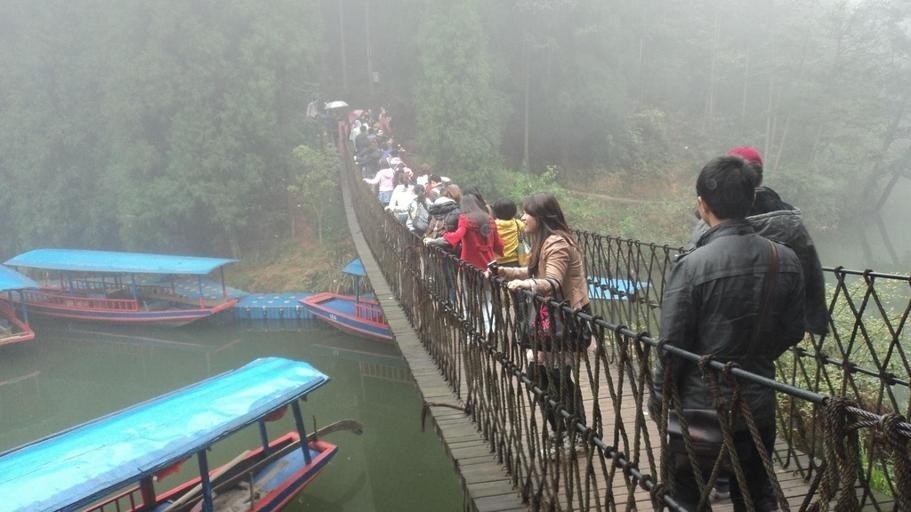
[540,431,592,462]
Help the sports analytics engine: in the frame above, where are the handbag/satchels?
[667,408,731,471]
[525,277,576,343]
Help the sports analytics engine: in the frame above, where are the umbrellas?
[323,99,348,109]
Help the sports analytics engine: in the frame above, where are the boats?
[1,356,361,511]
[1,248,240,327]
[297,258,652,344]
[14,314,243,353]
[1,265,40,346]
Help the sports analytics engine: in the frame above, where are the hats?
[726,146,763,168]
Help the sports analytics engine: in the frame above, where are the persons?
[688,144,830,494]
[489,196,526,268]
[485,192,594,458]
[422,196,504,348]
[647,154,807,512]
[346,106,494,241]
